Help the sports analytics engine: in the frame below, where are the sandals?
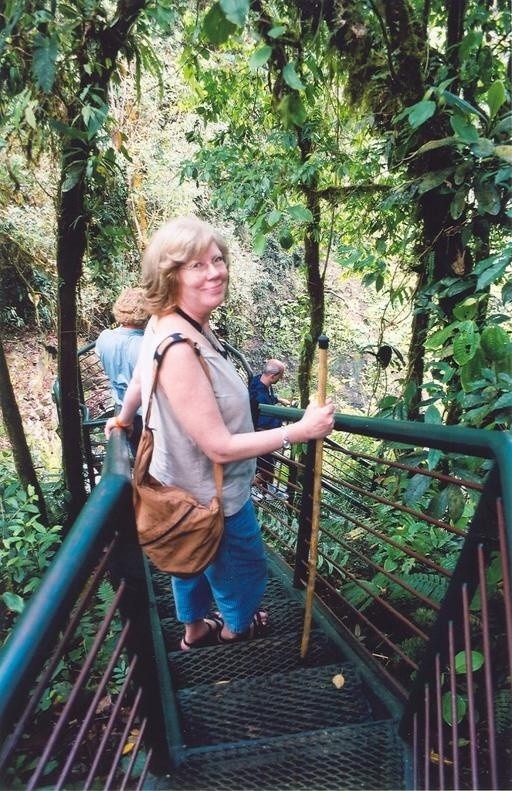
[179,608,226,653]
[217,611,269,644]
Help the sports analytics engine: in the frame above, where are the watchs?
[281,426,293,451]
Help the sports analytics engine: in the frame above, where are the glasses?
[179,254,231,273]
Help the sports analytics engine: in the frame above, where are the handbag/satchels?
[126,426,226,579]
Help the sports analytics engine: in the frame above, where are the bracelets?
[113,415,137,431]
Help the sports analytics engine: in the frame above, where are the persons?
[105,220,337,652]
[94,288,150,450]
[249,359,286,504]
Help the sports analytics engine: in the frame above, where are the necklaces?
[170,301,230,362]
